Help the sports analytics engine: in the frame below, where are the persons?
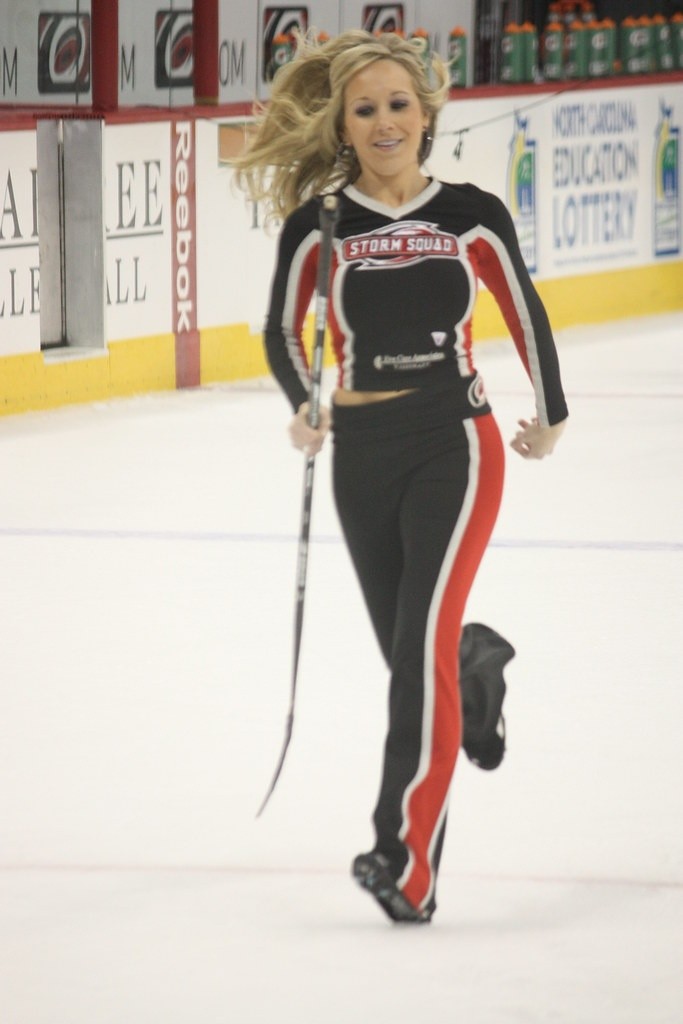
[209,29,570,924]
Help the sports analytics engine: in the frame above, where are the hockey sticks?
[252,192,340,819]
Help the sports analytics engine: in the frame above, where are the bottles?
[447,26,466,86]
[499,4,683,81]
[374,29,431,89]
[273,36,290,70]
[318,32,330,45]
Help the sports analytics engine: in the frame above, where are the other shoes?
[351,855,432,927]
[457,622,518,770]
